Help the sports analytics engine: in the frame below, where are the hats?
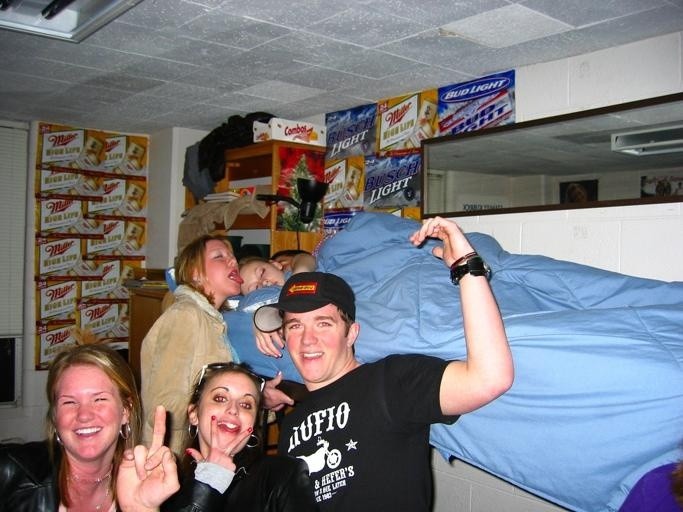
[253,272,356,333]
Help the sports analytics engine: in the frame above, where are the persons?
[253,250,317,358]
[236,256,286,296]
[563,184,588,202]
[163,361,320,511]
[138,233,297,458]
[116,403,180,512]
[0,343,138,512]
[257,216,516,511]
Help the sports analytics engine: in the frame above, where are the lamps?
[254,178,328,251]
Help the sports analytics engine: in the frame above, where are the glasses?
[194,363,267,397]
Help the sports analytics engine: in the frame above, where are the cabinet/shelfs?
[183,139,326,258]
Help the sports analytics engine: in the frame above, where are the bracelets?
[449,250,477,268]
[448,257,490,284]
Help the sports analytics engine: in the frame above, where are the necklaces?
[64,463,112,511]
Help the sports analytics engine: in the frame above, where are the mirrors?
[419,92,682,220]
